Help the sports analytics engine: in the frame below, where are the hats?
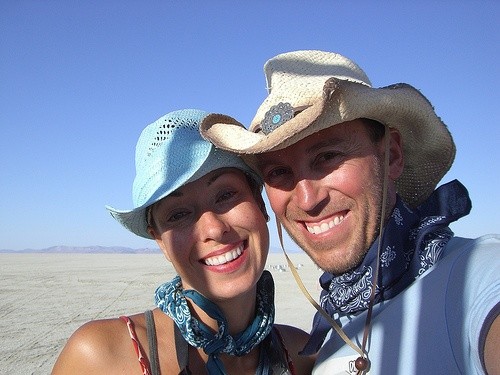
[105,109,263,240]
[199,49,456,206]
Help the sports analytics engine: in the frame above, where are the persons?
[247,49,500,375]
[50,108,323,375]
